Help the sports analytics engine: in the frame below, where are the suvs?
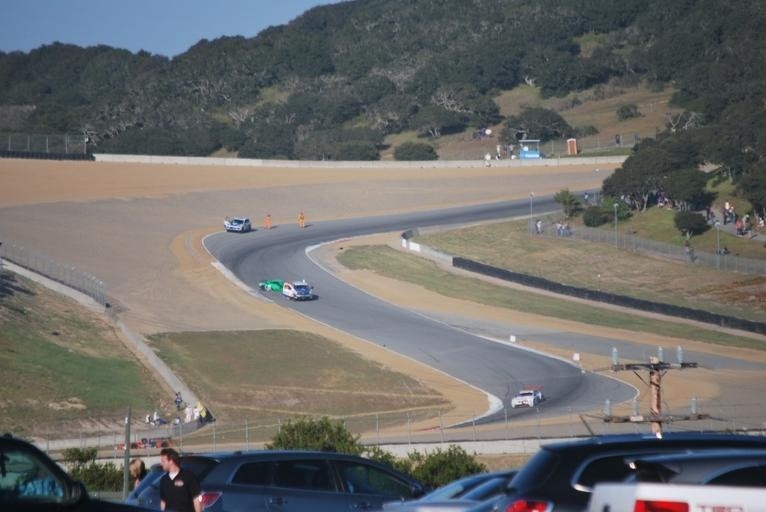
[224,215,252,233]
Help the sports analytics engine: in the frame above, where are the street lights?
[528,192,535,234]
[613,203,619,246]
[714,220,720,256]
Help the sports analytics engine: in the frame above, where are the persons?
[710,212,715,218]
[127,457,148,489]
[723,199,765,240]
[480,127,487,144]
[495,144,502,160]
[532,219,571,238]
[507,142,517,160]
[615,133,621,147]
[583,191,588,201]
[706,203,710,219]
[485,151,492,168]
[656,191,674,209]
[158,447,203,512]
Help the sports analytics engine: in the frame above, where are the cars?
[258,278,314,301]
[511,385,545,408]
